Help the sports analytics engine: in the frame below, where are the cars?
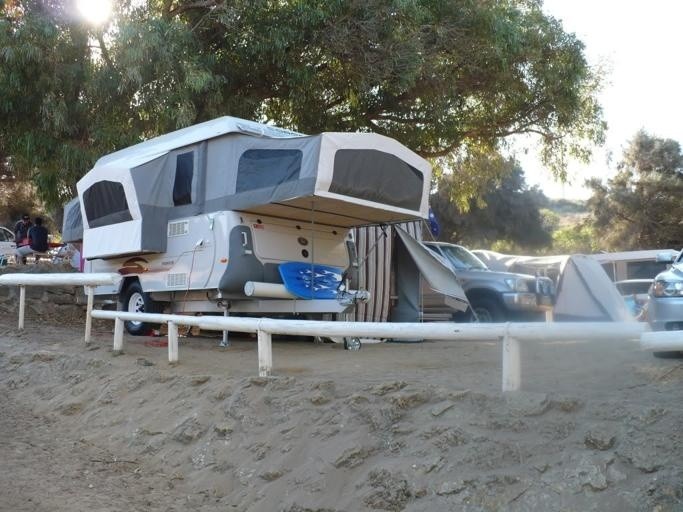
[614,247,683,331]
[422,240,556,322]
[0,227,17,255]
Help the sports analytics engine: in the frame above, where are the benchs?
[24,252,55,265]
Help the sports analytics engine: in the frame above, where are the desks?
[18,239,63,247]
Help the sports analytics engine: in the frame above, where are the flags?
[429,207,441,236]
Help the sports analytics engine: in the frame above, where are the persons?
[17,218,48,265]
[12,213,34,264]
